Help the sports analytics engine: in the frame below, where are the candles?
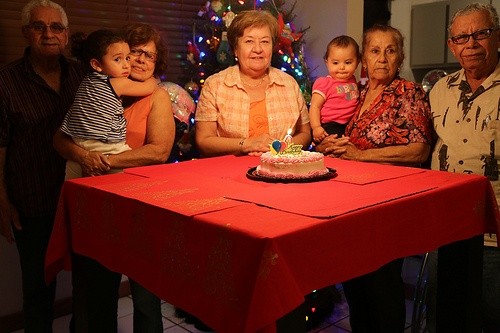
[287,128,292,154]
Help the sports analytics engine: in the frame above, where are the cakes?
[255,135,329,179]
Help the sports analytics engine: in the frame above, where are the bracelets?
[239,137,244,154]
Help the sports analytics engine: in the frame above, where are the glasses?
[450,29,498,44]
[29,23,65,34]
[130,48,159,63]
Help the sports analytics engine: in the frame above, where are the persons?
[0,0,86,333]
[428,4,500,333]
[309,34,360,144]
[195,10,310,156]
[309,26,430,163]
[51,21,176,333]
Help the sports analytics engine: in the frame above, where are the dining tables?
[43,153,500,333]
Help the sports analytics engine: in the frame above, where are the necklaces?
[239,75,267,88]
